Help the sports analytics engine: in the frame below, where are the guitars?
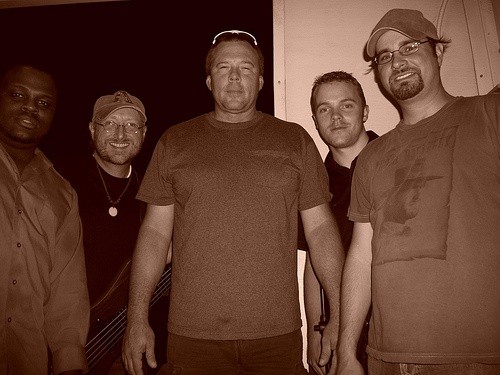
[84,254,172,375]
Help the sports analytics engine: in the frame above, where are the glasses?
[373,39,430,65]
[212,30,257,45]
[94,120,144,135]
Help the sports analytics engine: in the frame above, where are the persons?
[67,90,173,375]
[0,58,92,375]
[335,9,500,375]
[120,30,347,375]
[297,70,382,375]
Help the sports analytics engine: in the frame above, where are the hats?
[92,90,147,123]
[366,8,440,57]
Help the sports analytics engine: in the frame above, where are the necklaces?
[94,157,133,217]
